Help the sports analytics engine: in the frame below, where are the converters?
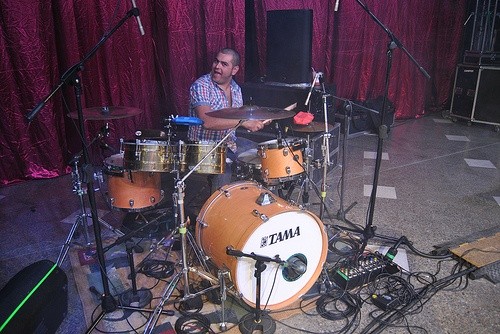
[373,293,400,311]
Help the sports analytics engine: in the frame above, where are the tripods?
[295,0,431,254]
[29,7,241,334]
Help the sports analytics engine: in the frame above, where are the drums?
[174,141,227,175]
[101,151,164,211]
[256,133,313,185]
[193,178,330,312]
[231,148,286,193]
[123,141,178,174]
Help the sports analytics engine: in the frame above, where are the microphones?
[334,0,339,12]
[131,0,145,35]
[288,257,307,273]
[305,72,318,106]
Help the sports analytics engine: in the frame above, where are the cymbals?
[67,104,144,121]
[206,105,298,120]
[284,123,331,133]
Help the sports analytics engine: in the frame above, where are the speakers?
[266,9,313,85]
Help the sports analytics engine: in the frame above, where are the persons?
[187,49,272,194]
